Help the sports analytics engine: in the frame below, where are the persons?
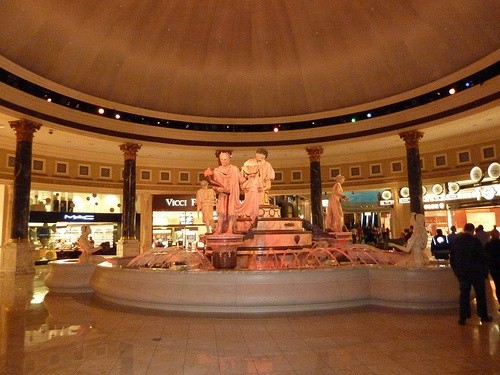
[347,223,413,249]
[89,236,94,248]
[389,212,431,269]
[212,152,245,235]
[325,174,349,232]
[431,222,500,326]
[243,148,275,205]
[78,224,103,263]
[195,177,216,234]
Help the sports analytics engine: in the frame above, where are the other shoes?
[458,320,466,325]
[477,312,490,321]
